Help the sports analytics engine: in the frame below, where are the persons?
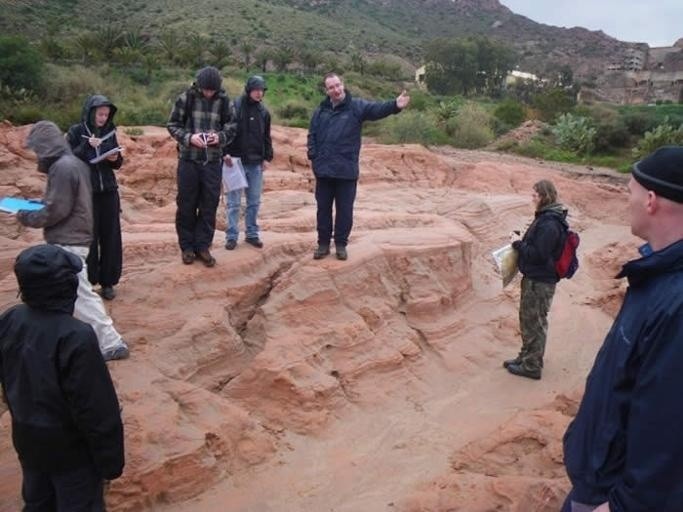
[219,74,274,250]
[305,71,411,260]
[0,118,129,362]
[0,243,126,510]
[501,180,571,380]
[65,94,126,301]
[558,144,682,512]
[165,66,239,268]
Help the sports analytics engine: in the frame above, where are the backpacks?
[545,215,579,279]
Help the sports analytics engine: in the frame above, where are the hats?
[630,146,682,202]
[196,67,221,90]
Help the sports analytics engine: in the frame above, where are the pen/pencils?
[81,135,91,139]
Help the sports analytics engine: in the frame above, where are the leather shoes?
[182,247,195,263]
[225,239,238,250]
[102,286,114,301]
[244,236,264,247]
[336,245,347,259]
[509,364,542,379]
[197,251,216,266]
[313,246,330,259]
[503,358,523,370]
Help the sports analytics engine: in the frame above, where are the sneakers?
[100,344,130,360]
